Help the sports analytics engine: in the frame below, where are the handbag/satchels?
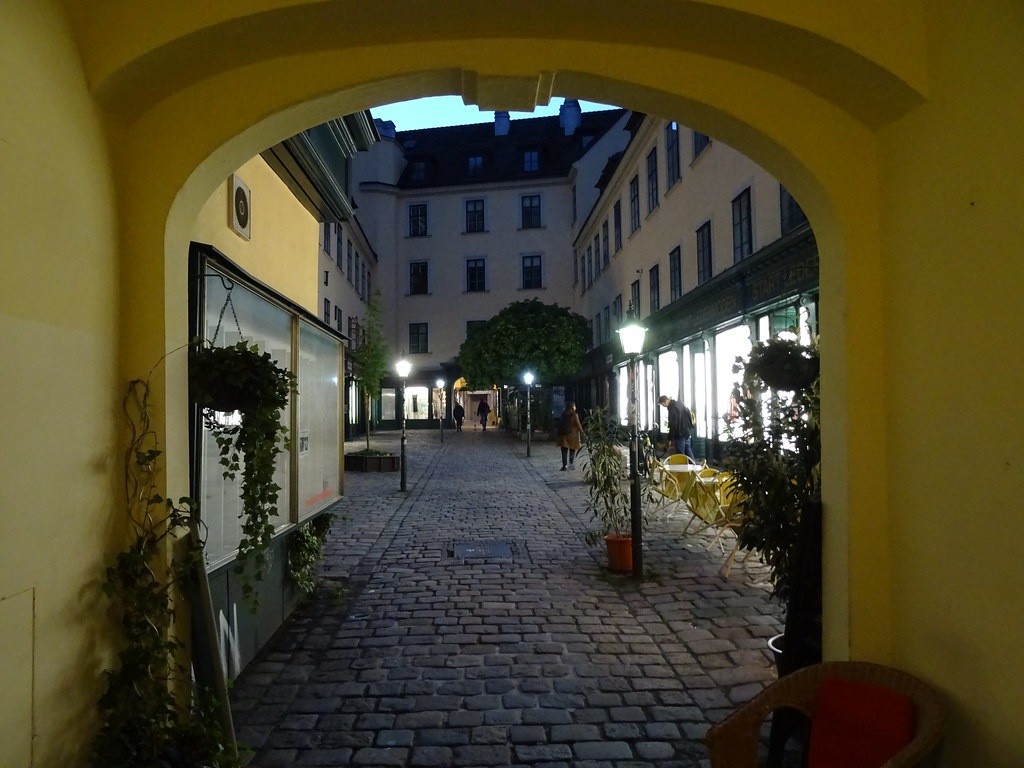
[687,407,696,428]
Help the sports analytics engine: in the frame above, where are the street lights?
[436,375,445,442]
[615,297,651,585]
[524,369,534,457]
[394,349,414,492]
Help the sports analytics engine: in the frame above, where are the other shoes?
[570,464,574,469]
[483,428,486,431]
[560,466,568,470]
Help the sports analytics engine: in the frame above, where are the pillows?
[807,672,917,768]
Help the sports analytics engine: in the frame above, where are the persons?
[453,402,464,432]
[556,402,582,471]
[658,395,696,465]
[477,399,491,432]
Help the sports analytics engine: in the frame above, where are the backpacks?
[557,410,576,435]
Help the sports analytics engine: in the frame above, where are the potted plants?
[572,404,656,572]
[183,335,297,610]
[740,340,821,469]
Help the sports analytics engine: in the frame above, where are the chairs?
[659,454,755,579]
[706,661,950,768]
